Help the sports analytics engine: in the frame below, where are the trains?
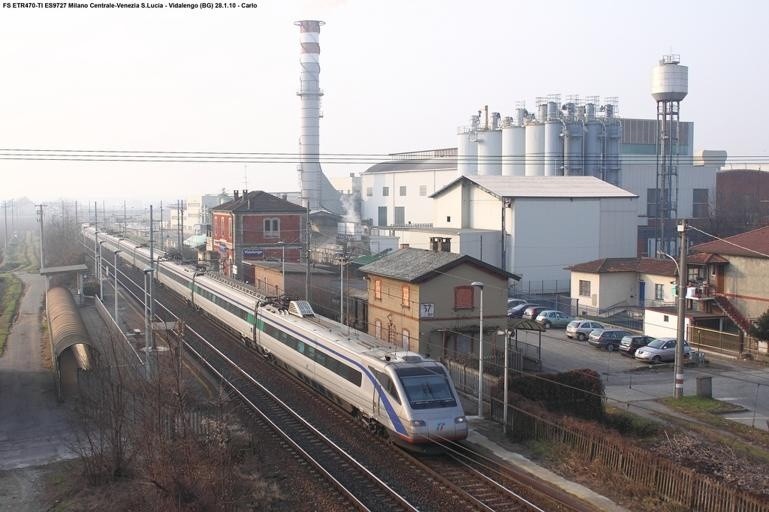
[81,223,469,457]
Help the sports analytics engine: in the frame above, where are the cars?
[535,310,577,328]
[635,336,689,362]
[566,319,605,340]
[508,298,552,320]
[619,335,657,354]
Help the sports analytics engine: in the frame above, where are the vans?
[588,328,635,350]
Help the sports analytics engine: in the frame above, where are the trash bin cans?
[696,375,712,398]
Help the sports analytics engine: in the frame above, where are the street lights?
[656,218,687,397]
[470,281,484,417]
